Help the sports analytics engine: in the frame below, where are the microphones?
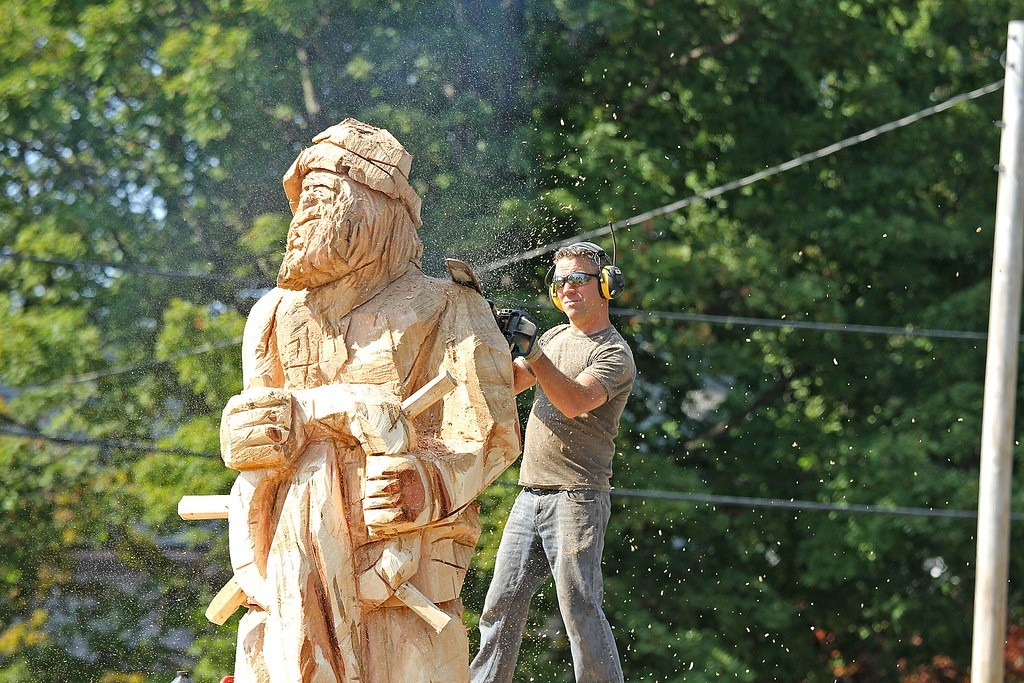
[608,220,617,265]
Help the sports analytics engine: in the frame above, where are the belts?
[524,487,560,495]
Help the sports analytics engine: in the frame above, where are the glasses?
[552,272,597,290]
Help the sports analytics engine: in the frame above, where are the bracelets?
[525,348,543,363]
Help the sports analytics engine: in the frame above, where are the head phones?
[543,241,626,312]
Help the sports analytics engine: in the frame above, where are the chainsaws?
[445,259,540,363]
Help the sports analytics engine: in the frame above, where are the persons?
[219,119,522,683]
[469,241,636,683]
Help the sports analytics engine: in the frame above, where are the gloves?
[497,310,543,364]
